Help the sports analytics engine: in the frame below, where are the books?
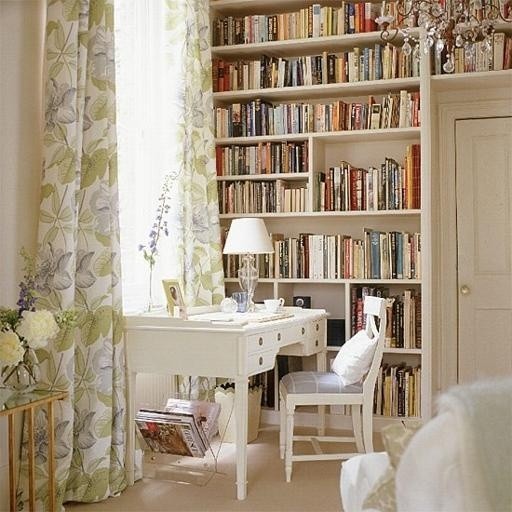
[318,143,421,211]
[217,140,310,213]
[222,227,422,279]
[350,285,422,349]
[132,397,219,459]
[363,358,421,418]
[214,1,420,137]
[247,355,304,408]
[432,0,511,74]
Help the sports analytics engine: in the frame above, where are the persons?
[169,284,183,305]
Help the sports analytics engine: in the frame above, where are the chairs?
[276,294,388,484]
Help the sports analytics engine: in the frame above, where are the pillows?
[329,329,379,387]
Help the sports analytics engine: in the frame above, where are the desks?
[125,303,333,501]
[0,386,69,512]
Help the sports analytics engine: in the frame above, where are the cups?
[232,292,247,314]
[264,298,284,309]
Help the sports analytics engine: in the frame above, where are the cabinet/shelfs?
[429,2,512,92]
[202,0,440,436]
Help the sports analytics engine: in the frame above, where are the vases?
[4,346,41,393]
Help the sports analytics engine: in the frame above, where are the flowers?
[0,306,62,383]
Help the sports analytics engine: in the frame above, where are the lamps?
[222,218,277,314]
[373,0,512,73]
